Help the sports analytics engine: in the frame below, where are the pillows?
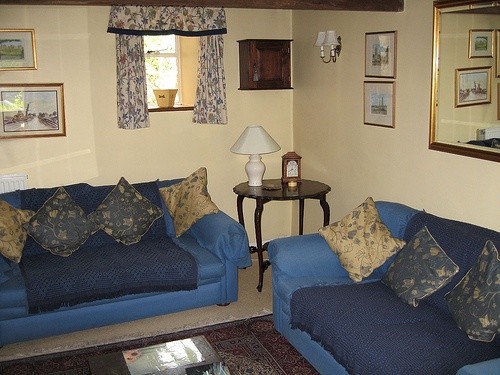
[319,197,500,342]
[0,167,218,263]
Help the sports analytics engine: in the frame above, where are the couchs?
[268,202,500,375]
[0,178,252,349]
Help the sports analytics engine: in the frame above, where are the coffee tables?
[88,336,229,375]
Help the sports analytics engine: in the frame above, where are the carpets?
[0,313,320,375]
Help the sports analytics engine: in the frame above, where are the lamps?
[314,30,342,63]
[230,126,280,186]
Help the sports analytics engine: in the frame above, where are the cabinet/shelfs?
[237,39,294,91]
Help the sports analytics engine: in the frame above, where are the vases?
[154,89,178,108]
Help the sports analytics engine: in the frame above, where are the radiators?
[0,175,28,194]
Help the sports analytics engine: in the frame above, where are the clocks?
[282,152,302,183]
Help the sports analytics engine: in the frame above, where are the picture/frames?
[0,28,38,70]
[495,29,500,77]
[363,81,396,128]
[0,83,66,139]
[455,66,492,107]
[365,31,397,78]
[468,29,495,58]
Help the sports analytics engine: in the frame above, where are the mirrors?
[428,0,500,163]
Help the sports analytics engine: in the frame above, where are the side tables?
[233,179,331,292]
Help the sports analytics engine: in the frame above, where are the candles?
[288,181,297,186]
